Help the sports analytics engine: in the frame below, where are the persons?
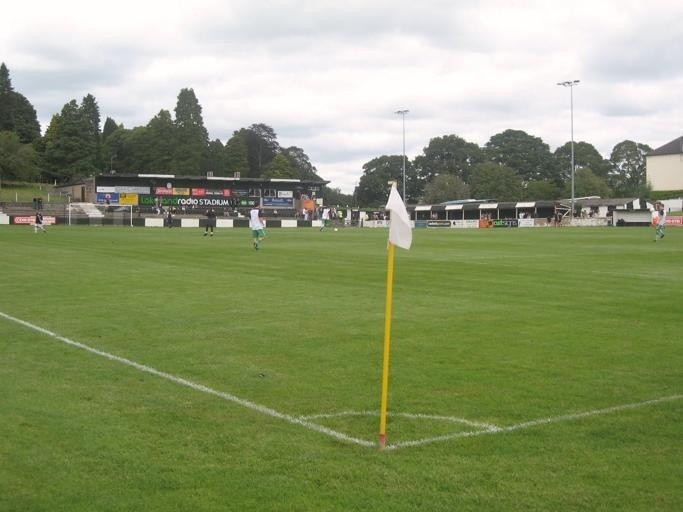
[542,212,564,230]
[204,206,217,237]
[168,212,171,223]
[581,210,598,218]
[33,196,42,209]
[651,202,666,241]
[106,196,110,204]
[304,208,309,221]
[320,206,331,232]
[35,208,47,234]
[249,202,265,250]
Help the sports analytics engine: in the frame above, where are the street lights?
[394,109,410,206]
[111,154,118,170]
[556,80,580,217]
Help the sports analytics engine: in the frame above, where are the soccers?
[334,228,338,231]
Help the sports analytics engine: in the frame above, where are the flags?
[385,184,415,250]
